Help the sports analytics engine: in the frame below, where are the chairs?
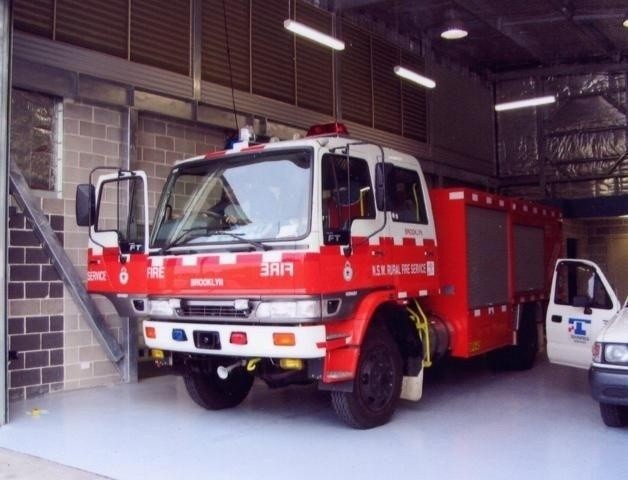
[328,181,367,229]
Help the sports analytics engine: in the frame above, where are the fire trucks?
[74,120,569,430]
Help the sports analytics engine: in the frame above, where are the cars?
[545,256,628,428]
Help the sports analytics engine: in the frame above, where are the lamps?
[440,8,469,41]
[394,65,436,90]
[283,0,345,54]
[494,95,556,112]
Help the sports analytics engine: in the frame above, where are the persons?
[200,181,252,230]
